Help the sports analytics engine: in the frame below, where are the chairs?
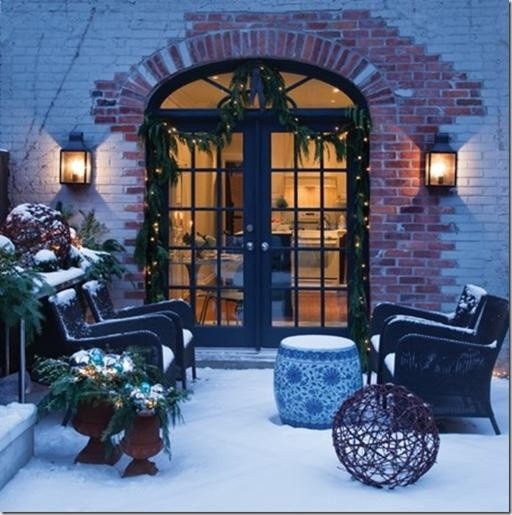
[195,261,244,326]
[367,284,509,435]
[48,278,197,427]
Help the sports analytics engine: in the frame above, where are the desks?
[170,255,238,325]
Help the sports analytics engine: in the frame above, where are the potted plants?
[30,346,197,478]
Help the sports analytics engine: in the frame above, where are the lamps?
[59,131,92,186]
[423,132,456,188]
